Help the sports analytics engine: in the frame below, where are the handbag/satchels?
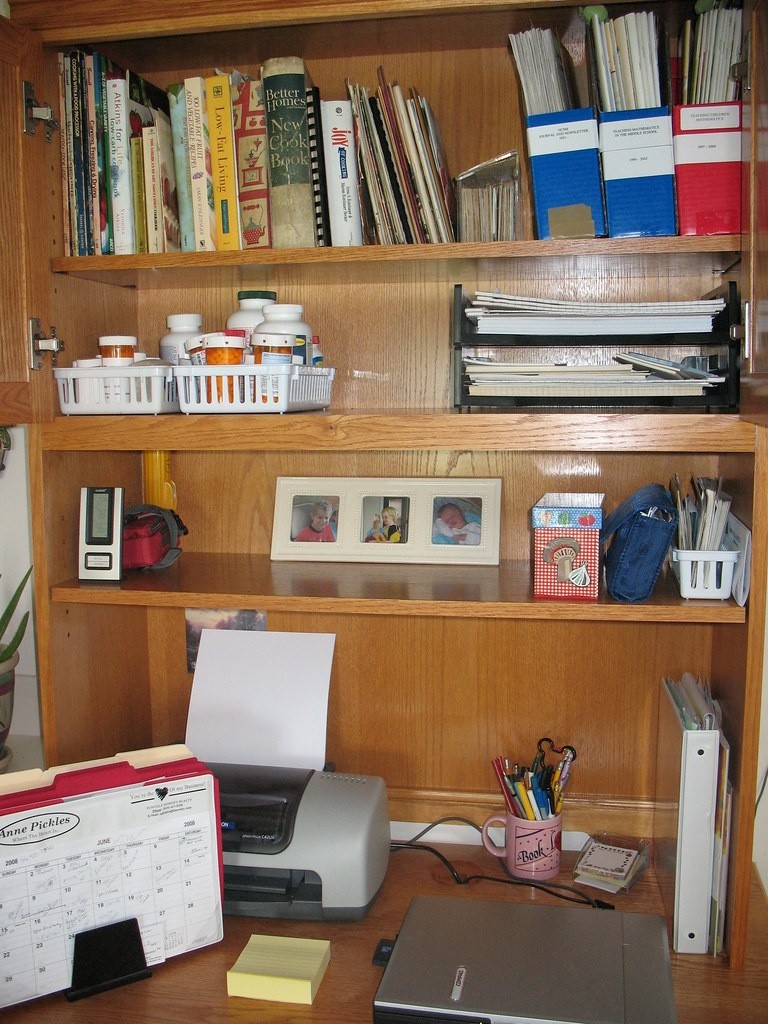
[598,484,680,602]
[121,504,189,574]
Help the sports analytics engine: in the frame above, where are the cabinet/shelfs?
[1,0,768,1024]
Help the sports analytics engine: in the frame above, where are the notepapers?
[575,842,644,888]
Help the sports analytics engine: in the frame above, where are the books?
[59,48,456,256]
[462,290,725,397]
[507,8,744,112]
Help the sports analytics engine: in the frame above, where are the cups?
[482,807,562,881]
[140,451,177,511]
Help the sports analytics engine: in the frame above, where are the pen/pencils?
[492,737,577,821]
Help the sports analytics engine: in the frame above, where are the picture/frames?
[268,476,504,568]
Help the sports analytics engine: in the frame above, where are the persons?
[366,508,400,542]
[293,500,335,541]
[433,504,480,545]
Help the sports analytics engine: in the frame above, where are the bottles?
[160,291,324,404]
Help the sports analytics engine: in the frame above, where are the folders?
[649,675,720,955]
